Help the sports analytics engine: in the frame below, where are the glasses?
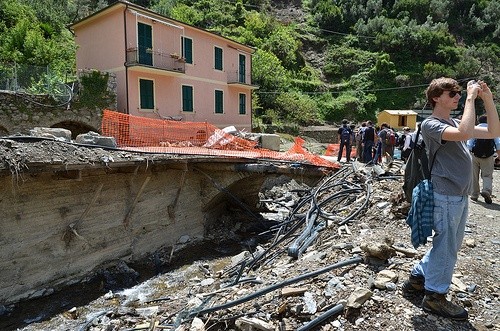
[449,90,462,99]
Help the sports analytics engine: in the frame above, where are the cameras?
[474,82,483,98]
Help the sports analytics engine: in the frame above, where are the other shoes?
[346,161,352,164]
[481,192,492,204]
[337,159,340,161]
[470,197,478,204]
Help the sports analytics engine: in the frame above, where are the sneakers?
[402,273,426,293]
[422,293,468,321]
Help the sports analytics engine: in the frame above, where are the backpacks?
[472,139,497,159]
[382,129,396,145]
[403,122,447,205]
[340,126,351,145]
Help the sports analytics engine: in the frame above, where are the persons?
[336,118,413,168]
[401,77,500,322]
[466,114,500,204]
